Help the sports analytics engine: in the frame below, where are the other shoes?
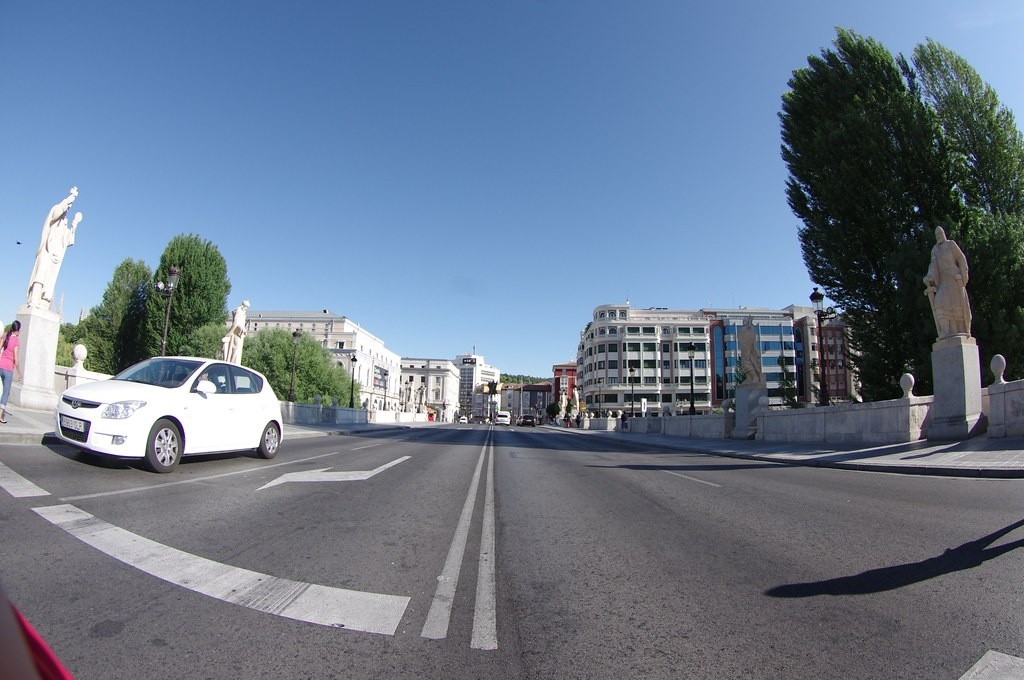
[0,418,7,423]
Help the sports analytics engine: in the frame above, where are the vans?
[495,412,510,425]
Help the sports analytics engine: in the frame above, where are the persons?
[221,298,250,366]
[565,413,572,427]
[406,380,427,405]
[557,387,580,420]
[923,227,972,341]
[621,410,628,432]
[193,369,217,393]
[26,187,83,312]
[0,320,22,423]
[577,413,581,428]
[738,316,765,385]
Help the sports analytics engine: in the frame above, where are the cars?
[55,356,283,474]
[460,416,467,423]
[516,414,536,426]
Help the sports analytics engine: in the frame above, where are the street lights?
[383,370,389,410]
[153,265,181,356]
[289,329,303,402]
[404,381,409,411]
[810,286,831,406]
[597,378,603,418]
[688,344,696,415]
[629,366,636,418]
[349,356,358,408]
